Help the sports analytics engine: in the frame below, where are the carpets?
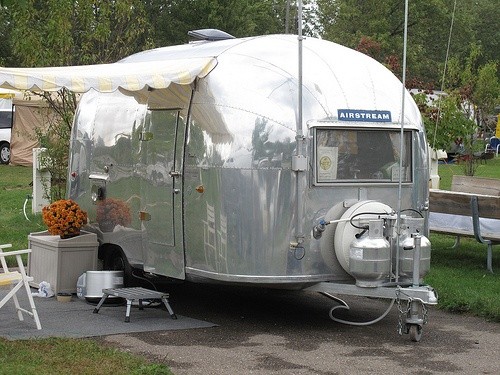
[0,267,220,342]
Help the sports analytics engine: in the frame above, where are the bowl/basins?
[56,292,72,302]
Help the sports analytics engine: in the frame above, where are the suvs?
[0,109,15,164]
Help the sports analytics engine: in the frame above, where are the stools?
[92,287,179,323]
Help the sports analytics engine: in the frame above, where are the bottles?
[77,271,87,301]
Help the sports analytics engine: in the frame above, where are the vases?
[60,234,79,239]
[99,227,115,232]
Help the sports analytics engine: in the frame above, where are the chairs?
[0,243,41,330]
[485,136,500,157]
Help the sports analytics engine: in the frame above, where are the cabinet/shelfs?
[27,228,99,297]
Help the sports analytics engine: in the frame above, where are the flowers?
[95,198,131,228]
[41,198,88,236]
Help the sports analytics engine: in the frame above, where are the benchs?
[450,176,500,250]
[429,190,500,274]
